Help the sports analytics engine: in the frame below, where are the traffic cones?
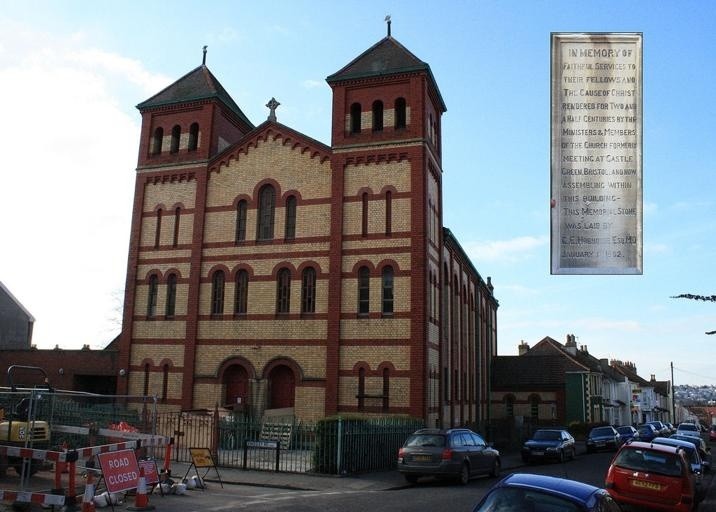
[80,471,98,511]
[126,467,156,512]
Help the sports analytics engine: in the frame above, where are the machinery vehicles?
[0,408,49,478]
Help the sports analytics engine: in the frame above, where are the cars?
[521,430,576,463]
[710,425,716,441]
[676,423,702,437]
[636,434,712,485]
[637,420,673,441]
[472,473,624,512]
[586,426,623,453]
[616,425,638,444]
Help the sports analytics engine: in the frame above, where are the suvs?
[605,441,698,511]
[397,428,501,484]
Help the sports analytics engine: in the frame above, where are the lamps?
[120,369,126,377]
[58,368,64,375]
